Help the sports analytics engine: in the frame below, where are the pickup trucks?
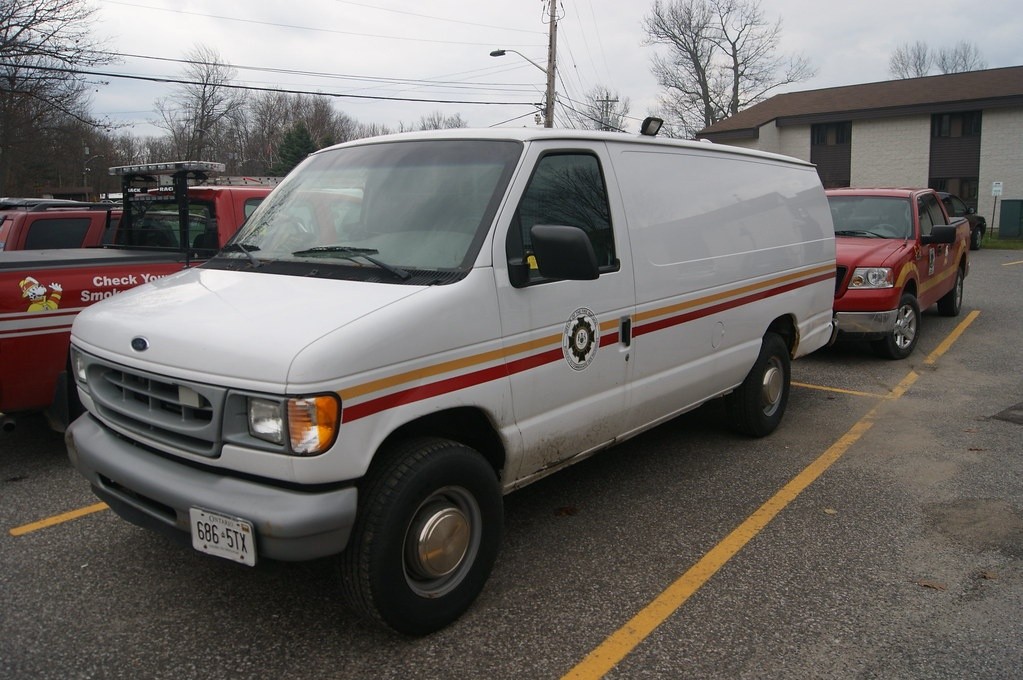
[0,161,365,435]
[825,187,972,360]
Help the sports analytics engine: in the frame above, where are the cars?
[936,192,986,251]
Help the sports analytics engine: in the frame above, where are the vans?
[64,128,840,641]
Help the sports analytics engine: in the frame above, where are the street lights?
[489,49,557,127]
